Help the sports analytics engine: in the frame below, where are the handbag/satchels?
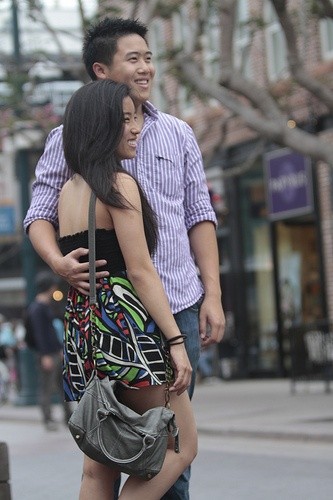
[65,371,182,481]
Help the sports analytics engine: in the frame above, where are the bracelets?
[166,334,184,344]
[167,340,185,346]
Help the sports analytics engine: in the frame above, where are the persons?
[56,77,198,500]
[22,17,225,500]
[26,271,72,430]
